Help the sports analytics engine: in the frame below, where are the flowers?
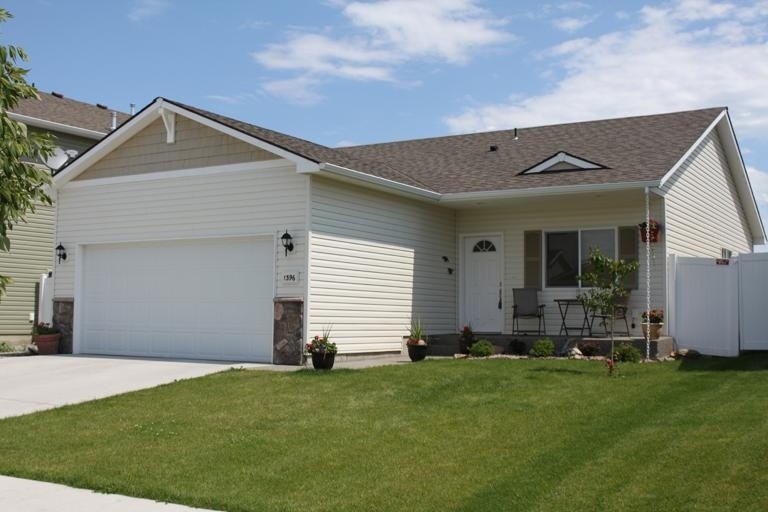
[405,313,428,345]
[458,326,476,338]
[33,322,63,334]
[305,322,337,352]
[638,219,661,229]
[642,310,664,322]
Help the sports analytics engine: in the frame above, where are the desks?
[553,298,595,338]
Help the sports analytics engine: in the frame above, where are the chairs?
[589,287,632,337]
[511,287,546,337]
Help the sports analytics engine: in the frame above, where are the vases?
[459,338,477,354]
[406,344,427,362]
[640,229,659,242]
[311,352,335,369]
[642,322,664,338]
[33,334,60,355]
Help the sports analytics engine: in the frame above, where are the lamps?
[281,229,293,257]
[55,241,67,264]
